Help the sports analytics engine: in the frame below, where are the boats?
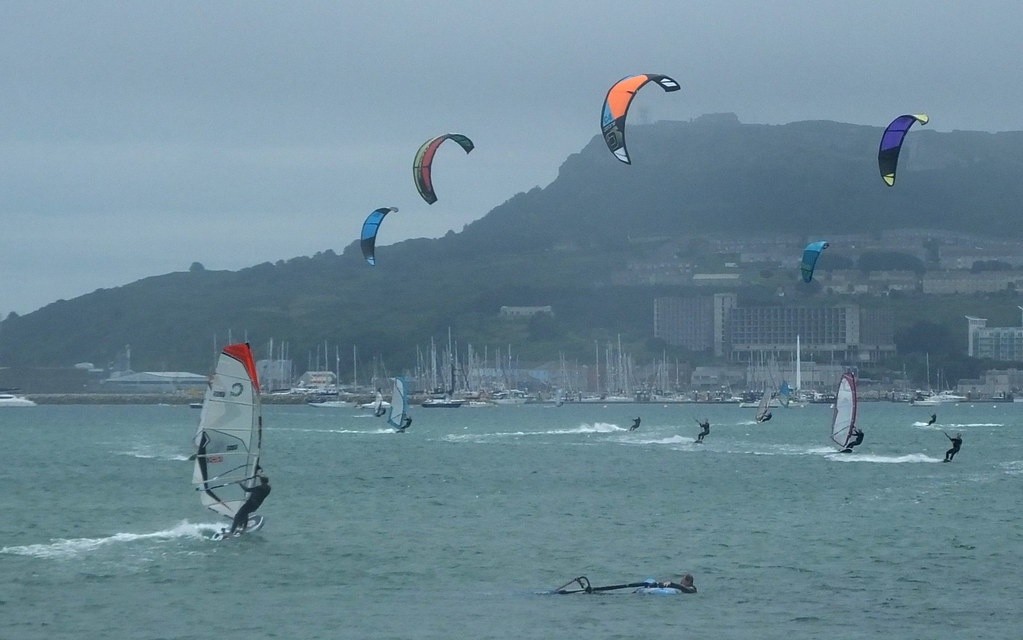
[310,399,361,409]
[364,400,392,409]
[468,402,495,410]
[421,398,463,407]
[0,393,40,408]
[491,396,530,406]
[937,390,969,403]
[911,397,943,406]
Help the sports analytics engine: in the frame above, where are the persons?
[664,573,698,593]
[943,432,963,460]
[629,416,641,431]
[927,412,936,425]
[762,412,772,422]
[696,418,710,441]
[845,428,864,449]
[401,416,413,429]
[223,475,272,536]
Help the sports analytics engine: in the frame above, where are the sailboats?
[187,340,271,540]
[387,378,413,435]
[832,372,859,457]
